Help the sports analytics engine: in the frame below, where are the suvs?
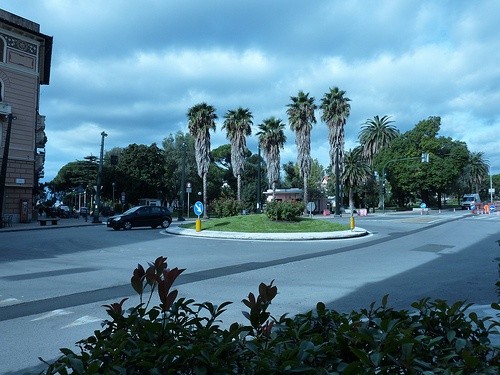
[36,199,70,218]
[107,205,172,230]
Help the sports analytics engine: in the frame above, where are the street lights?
[93,132,108,223]
[489,165,493,203]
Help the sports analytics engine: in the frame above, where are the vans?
[460,194,481,209]
